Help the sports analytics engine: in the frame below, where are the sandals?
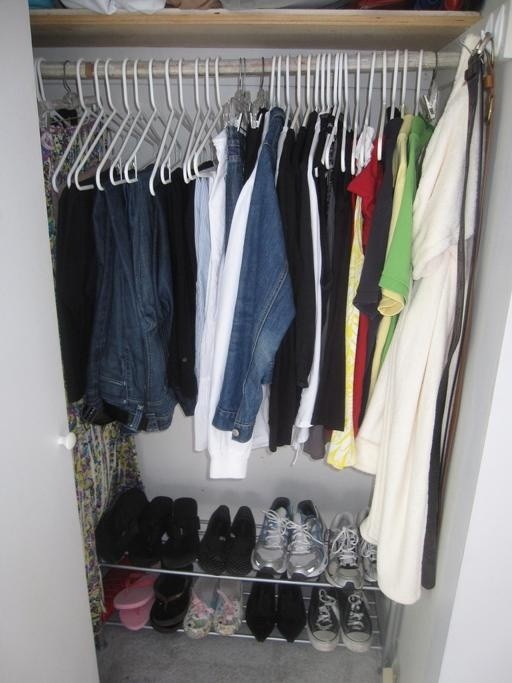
[183,576,215,640]
[132,495,172,566]
[162,498,200,569]
[214,578,243,635]
[95,490,146,563]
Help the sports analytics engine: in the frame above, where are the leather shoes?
[277,575,307,643]
[247,572,276,643]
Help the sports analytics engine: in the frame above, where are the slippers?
[151,571,188,634]
[113,569,155,632]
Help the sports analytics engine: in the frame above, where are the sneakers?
[327,508,360,591]
[251,496,286,577]
[340,583,373,655]
[357,506,385,581]
[200,505,230,575]
[230,506,255,578]
[308,586,341,652]
[289,499,329,580]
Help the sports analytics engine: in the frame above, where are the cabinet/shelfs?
[92,520,399,671]
